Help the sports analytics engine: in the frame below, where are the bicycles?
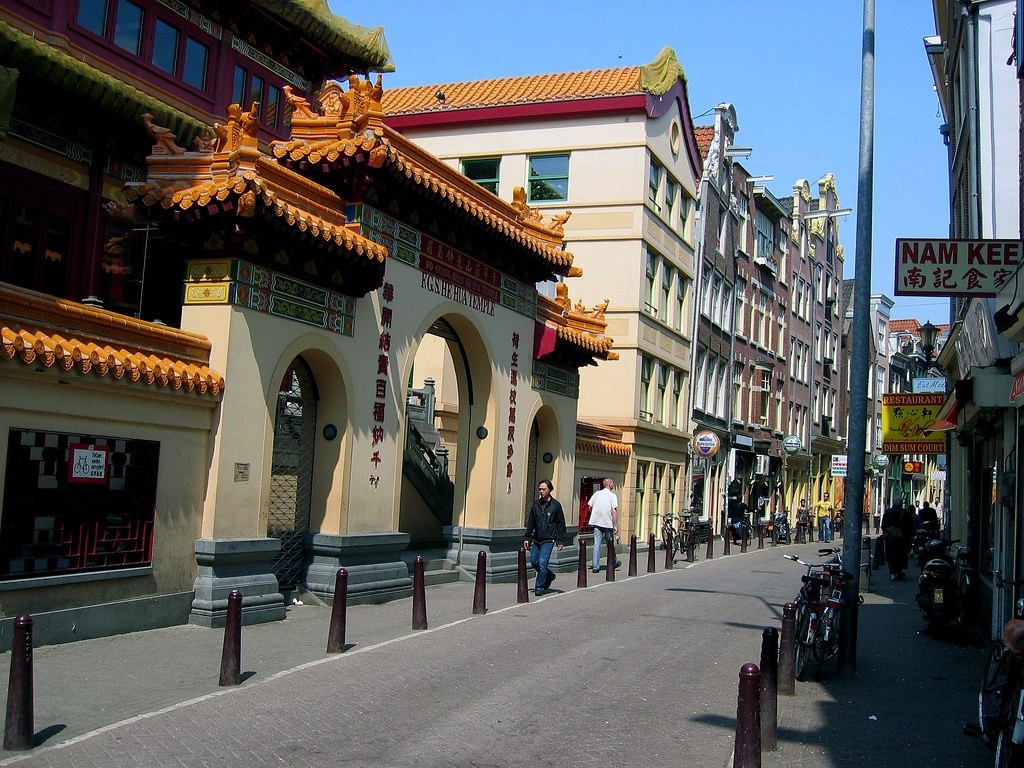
[950,542,978,627]
[978,599,1024,768]
[652,512,701,561]
[781,547,856,681]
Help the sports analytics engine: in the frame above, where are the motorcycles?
[768,507,793,542]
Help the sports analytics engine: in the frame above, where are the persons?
[881,496,913,581]
[918,501,938,530]
[731,495,754,546]
[814,492,832,543]
[909,505,922,559]
[587,478,621,573]
[931,497,942,529]
[794,499,809,544]
[523,479,566,596]
[915,501,921,515]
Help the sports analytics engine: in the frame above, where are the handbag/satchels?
[882,527,902,540]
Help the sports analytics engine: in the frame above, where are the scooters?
[732,510,759,542]
[796,512,818,533]
[911,518,945,552]
[914,539,959,634]
[834,507,846,532]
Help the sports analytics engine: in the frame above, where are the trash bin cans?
[859,535,872,592]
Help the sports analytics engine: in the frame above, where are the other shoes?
[592,567,600,573]
[535,588,544,596]
[891,574,896,580]
[544,573,556,589]
[615,560,621,569]
[897,572,905,579]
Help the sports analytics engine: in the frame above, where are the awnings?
[923,381,972,436]
